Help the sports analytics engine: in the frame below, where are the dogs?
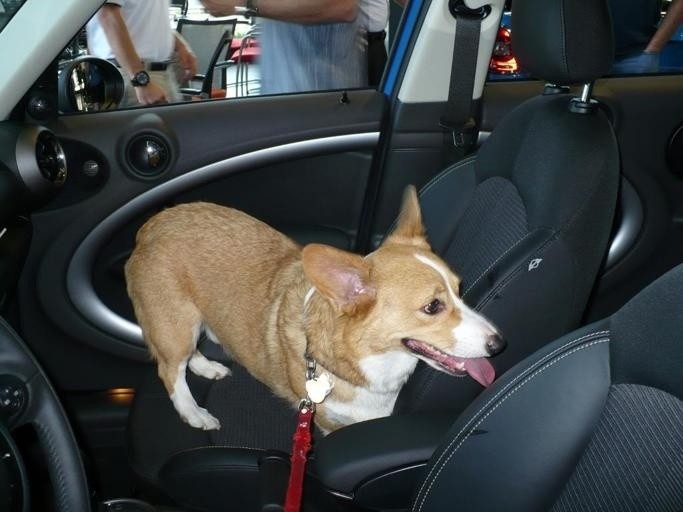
[121,182,509,437]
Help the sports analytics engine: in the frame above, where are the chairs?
[414,265,679,509]
[146,94,624,497]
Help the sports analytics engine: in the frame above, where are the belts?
[106,57,169,71]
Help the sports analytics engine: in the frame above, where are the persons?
[83,0,196,112]
[198,0,369,95]
[357,0,389,88]
[610,0,682,74]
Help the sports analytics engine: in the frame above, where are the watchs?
[244,0,257,18]
[130,70,149,87]
